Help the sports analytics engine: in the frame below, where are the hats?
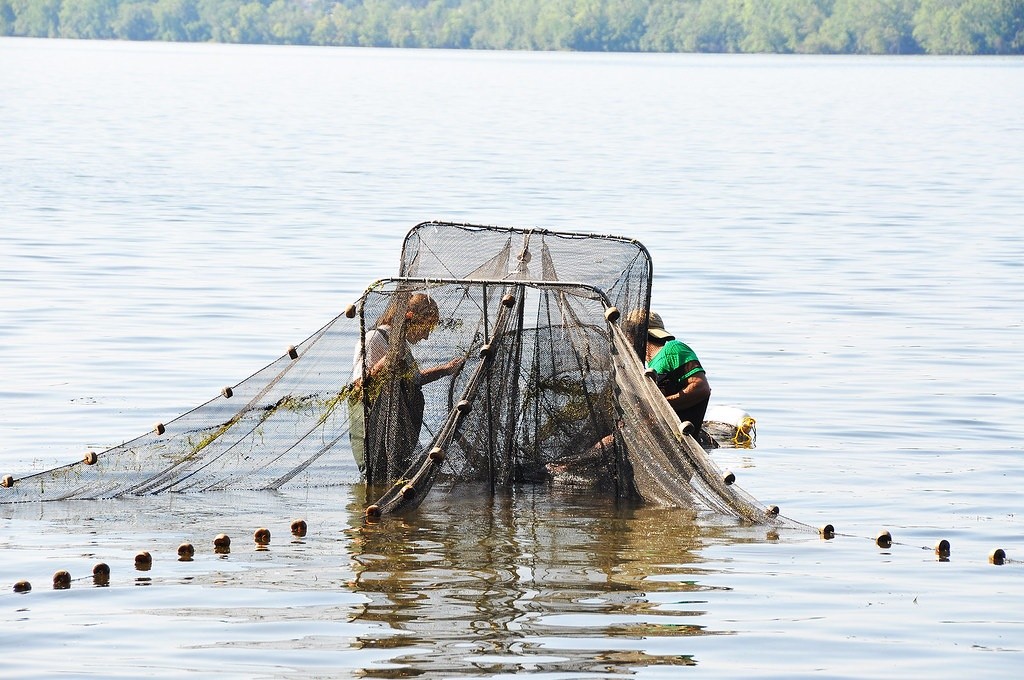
[624,308,676,339]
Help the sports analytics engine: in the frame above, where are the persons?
[348,293,464,474]
[547,310,710,478]
